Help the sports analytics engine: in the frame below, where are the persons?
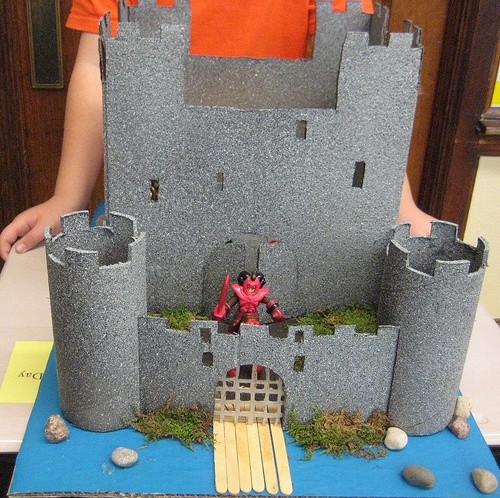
[1,0,445,265]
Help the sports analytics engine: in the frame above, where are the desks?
[2,235,500,453]
